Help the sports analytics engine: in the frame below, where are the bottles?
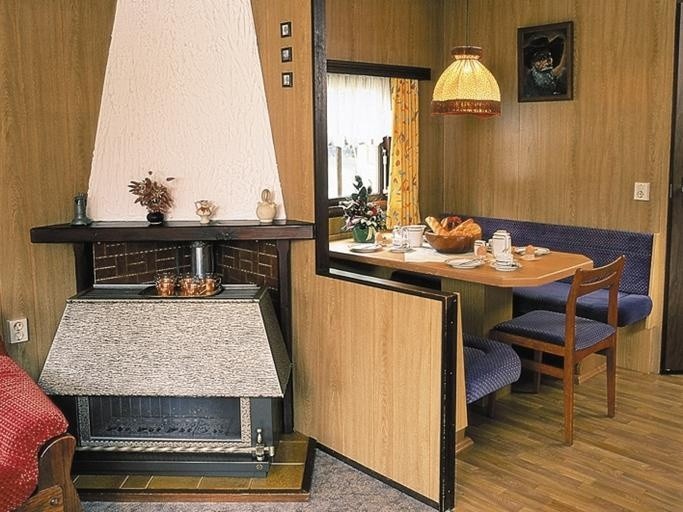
[70,193,92,225]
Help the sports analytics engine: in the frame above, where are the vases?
[351,224,375,243]
[146,210,164,225]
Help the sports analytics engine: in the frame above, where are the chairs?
[487,255,626,446]
[0,338,83,512]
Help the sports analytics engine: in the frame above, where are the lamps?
[430,0,501,120]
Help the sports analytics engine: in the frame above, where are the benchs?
[455,292,522,455]
[437,207,658,385]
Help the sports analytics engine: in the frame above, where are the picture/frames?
[280,47,292,62]
[281,72,293,87]
[516,20,575,103]
[279,21,291,38]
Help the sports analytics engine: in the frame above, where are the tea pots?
[194,201,217,224]
[255,190,276,224]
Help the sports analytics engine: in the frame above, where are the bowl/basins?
[424,229,482,254]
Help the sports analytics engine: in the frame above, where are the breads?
[424,216,482,238]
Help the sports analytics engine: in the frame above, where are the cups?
[152,271,221,296]
[490,230,512,260]
[389,223,426,248]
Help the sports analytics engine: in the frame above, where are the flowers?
[127,170,175,213]
[337,173,389,240]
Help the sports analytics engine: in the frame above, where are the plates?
[443,239,550,272]
[347,243,412,253]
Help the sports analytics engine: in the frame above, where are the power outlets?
[6,317,29,344]
